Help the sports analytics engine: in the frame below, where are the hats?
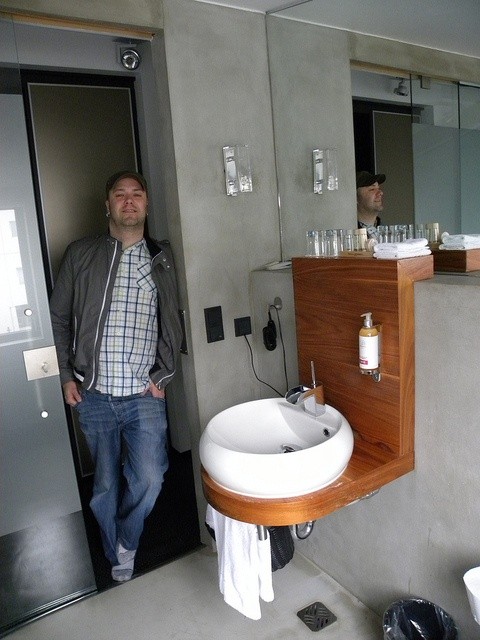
[357,170,386,186]
[106,170,146,194]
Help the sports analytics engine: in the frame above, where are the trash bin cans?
[382,597,459,640]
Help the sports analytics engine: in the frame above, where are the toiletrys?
[358,312,382,372]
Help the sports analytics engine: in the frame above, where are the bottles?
[305,230,318,256]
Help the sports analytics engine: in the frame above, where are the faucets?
[286,384,326,416]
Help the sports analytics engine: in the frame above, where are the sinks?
[198,398,355,499]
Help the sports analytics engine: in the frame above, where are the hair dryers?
[263,317,277,351]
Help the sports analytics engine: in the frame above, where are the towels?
[439,233,480,251]
[373,238,432,259]
[205,504,275,620]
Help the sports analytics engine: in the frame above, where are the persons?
[49,171,184,582]
[356,170,383,228]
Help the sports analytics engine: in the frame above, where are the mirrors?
[265,0,480,276]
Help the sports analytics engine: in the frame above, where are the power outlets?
[234,316,252,336]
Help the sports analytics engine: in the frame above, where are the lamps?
[394,81,409,97]
[115,43,141,71]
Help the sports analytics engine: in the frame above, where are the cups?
[359,228,368,249]
[376,225,387,246]
[367,239,377,250]
[325,230,338,256]
[426,224,431,243]
[344,230,353,250]
[318,230,326,257]
[353,228,361,251]
[432,222,440,244]
[390,225,401,245]
[406,224,414,241]
[395,224,408,242]
[337,230,343,254]
[417,224,426,239]
